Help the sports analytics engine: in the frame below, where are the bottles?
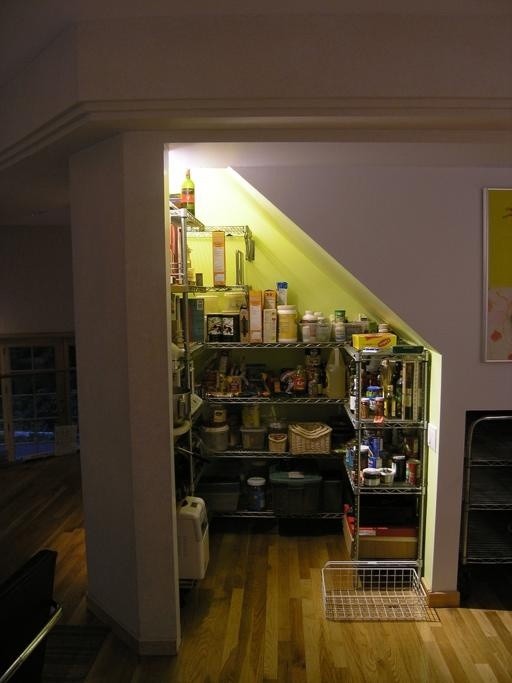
[182,169,195,217]
[349,371,360,414]
[308,373,323,398]
[392,359,412,419]
[275,304,299,344]
[302,309,346,342]
[360,383,396,420]
[378,323,388,332]
[293,364,306,396]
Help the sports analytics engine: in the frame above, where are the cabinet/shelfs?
[169,200,431,591]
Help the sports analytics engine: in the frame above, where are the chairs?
[0,549,63,683]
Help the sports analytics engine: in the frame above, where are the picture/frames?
[482,186,512,363]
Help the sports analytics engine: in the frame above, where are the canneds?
[213,404,227,427]
[406,459,421,486]
[345,436,393,488]
[392,456,405,482]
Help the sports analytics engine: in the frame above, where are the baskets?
[321,568,428,621]
[287,422,332,456]
[268,432,287,451]
